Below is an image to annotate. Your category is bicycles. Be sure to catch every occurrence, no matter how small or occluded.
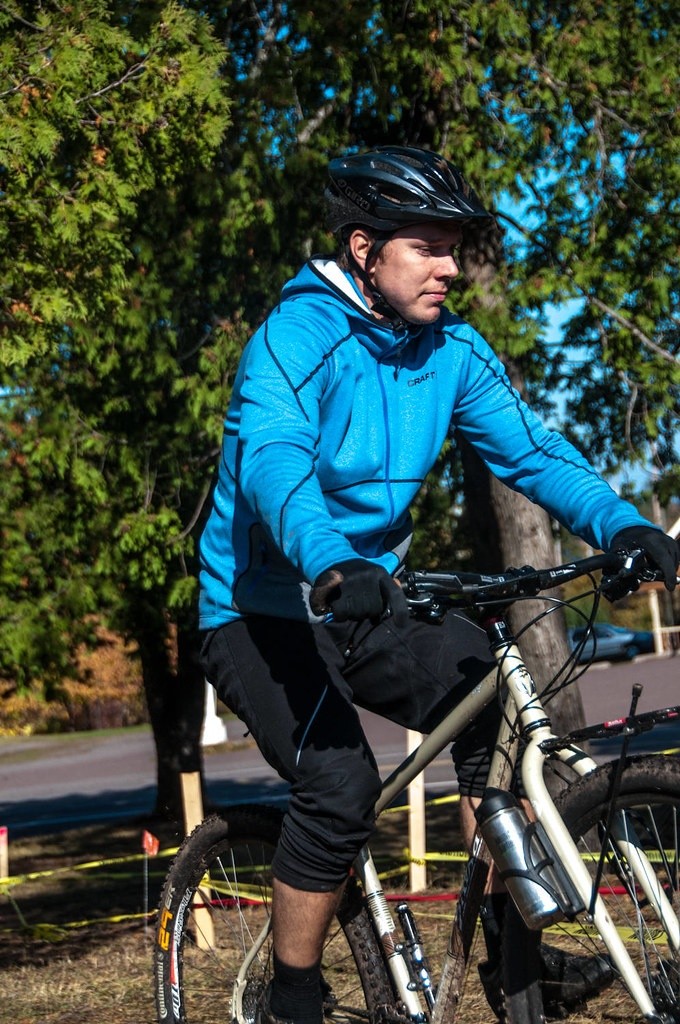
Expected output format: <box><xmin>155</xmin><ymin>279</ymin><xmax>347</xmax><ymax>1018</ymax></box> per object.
<box><xmin>154</xmin><ymin>540</ymin><xmax>680</xmax><ymax>1023</ymax></box>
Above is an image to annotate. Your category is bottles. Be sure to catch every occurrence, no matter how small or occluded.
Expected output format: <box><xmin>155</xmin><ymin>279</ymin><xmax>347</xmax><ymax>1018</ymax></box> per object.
<box><xmin>474</xmin><ymin>787</ymin><xmax>565</xmax><ymax>930</ymax></box>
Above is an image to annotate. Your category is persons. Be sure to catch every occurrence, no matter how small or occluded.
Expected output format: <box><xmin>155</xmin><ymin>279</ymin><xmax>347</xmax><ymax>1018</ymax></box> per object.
<box><xmin>197</xmin><ymin>147</ymin><xmax>680</xmax><ymax>1024</ymax></box>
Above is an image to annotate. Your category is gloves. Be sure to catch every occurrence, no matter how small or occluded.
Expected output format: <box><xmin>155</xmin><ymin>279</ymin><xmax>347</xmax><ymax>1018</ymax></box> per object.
<box><xmin>325</xmin><ymin>558</ymin><xmax>408</xmax><ymax>629</ymax></box>
<box><xmin>603</xmin><ymin>527</ymin><xmax>680</xmax><ymax>593</ymax></box>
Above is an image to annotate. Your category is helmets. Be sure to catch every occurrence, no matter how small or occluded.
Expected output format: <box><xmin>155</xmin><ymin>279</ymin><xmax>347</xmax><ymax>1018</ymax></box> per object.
<box><xmin>326</xmin><ymin>146</ymin><xmax>494</xmax><ymax>238</ymax></box>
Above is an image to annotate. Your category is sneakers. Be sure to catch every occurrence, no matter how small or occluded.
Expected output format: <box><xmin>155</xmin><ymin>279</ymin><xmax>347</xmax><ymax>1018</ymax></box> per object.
<box><xmin>478</xmin><ymin>943</ymin><xmax>615</xmax><ymax>1024</ymax></box>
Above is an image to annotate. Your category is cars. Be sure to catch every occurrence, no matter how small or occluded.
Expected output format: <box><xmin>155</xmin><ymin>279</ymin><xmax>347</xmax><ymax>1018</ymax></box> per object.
<box><xmin>567</xmin><ymin>622</ymin><xmax>654</xmax><ymax>665</ymax></box>
